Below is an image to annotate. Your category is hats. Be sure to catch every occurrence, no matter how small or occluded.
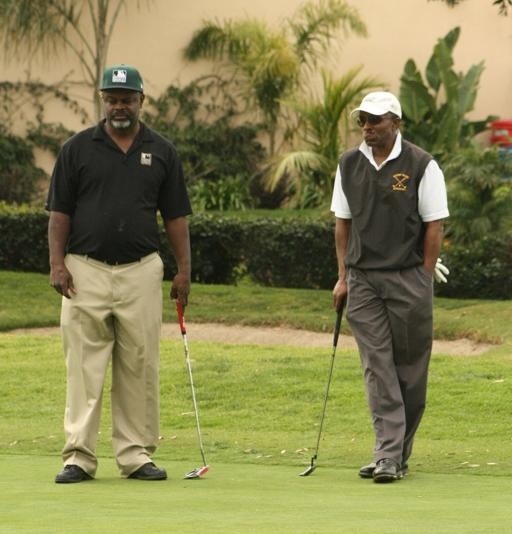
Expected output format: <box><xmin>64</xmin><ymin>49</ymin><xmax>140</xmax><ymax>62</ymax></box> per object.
<box><xmin>100</xmin><ymin>64</ymin><xmax>143</xmax><ymax>94</ymax></box>
<box><xmin>350</xmin><ymin>90</ymin><xmax>402</xmax><ymax>120</ymax></box>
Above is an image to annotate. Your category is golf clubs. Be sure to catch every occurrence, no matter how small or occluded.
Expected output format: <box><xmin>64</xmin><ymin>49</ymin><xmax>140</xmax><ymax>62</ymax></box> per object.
<box><xmin>176</xmin><ymin>297</ymin><xmax>209</xmax><ymax>479</ymax></box>
<box><xmin>300</xmin><ymin>294</ymin><xmax>345</xmax><ymax>476</ymax></box>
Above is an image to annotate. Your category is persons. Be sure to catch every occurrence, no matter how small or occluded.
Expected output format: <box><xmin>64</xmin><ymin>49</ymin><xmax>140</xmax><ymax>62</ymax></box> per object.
<box><xmin>44</xmin><ymin>63</ymin><xmax>193</xmax><ymax>484</ymax></box>
<box><xmin>329</xmin><ymin>91</ymin><xmax>450</xmax><ymax>484</ymax></box>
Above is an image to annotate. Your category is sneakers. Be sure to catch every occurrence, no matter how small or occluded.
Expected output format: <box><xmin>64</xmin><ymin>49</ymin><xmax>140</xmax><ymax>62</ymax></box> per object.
<box><xmin>359</xmin><ymin>463</ymin><xmax>408</xmax><ymax>478</ymax></box>
<box><xmin>373</xmin><ymin>459</ymin><xmax>405</xmax><ymax>482</ymax></box>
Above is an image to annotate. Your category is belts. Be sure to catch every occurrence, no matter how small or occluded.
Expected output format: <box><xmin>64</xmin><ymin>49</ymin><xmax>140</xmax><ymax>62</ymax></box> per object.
<box><xmin>84</xmin><ymin>249</ymin><xmax>161</xmax><ymax>267</ymax></box>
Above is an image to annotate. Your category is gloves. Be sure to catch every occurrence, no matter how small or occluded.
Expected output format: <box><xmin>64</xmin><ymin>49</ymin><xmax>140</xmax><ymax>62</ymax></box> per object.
<box><xmin>433</xmin><ymin>257</ymin><xmax>449</xmax><ymax>285</ymax></box>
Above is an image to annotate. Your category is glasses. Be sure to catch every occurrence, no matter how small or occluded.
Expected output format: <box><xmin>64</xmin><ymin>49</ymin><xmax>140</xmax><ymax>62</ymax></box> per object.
<box><xmin>356</xmin><ymin>113</ymin><xmax>394</xmax><ymax>126</ymax></box>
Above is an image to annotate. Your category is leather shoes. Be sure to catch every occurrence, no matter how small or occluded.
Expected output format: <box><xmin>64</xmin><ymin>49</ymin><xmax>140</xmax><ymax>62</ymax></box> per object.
<box><xmin>54</xmin><ymin>465</ymin><xmax>94</xmax><ymax>484</ymax></box>
<box><xmin>127</xmin><ymin>462</ymin><xmax>167</xmax><ymax>480</ymax></box>
